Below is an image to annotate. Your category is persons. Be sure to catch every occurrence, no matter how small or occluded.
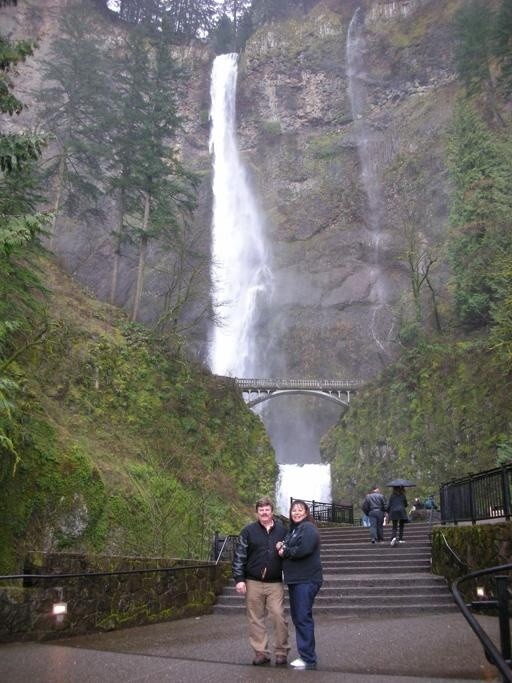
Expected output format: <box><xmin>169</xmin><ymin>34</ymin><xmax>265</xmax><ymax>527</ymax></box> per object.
<box><xmin>274</xmin><ymin>498</ymin><xmax>324</xmax><ymax>667</ymax></box>
<box><xmin>360</xmin><ymin>485</ymin><xmax>387</xmax><ymax>544</ymax></box>
<box><xmin>231</xmin><ymin>495</ymin><xmax>290</xmax><ymax>666</ymax></box>
<box><xmin>384</xmin><ymin>485</ymin><xmax>410</xmax><ymax>547</ymax></box>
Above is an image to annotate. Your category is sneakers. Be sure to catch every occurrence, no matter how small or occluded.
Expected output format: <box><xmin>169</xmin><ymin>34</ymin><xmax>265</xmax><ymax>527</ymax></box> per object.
<box><xmin>252</xmin><ymin>652</ymin><xmax>271</xmax><ymax>666</ymax></box>
<box><xmin>372</xmin><ymin>537</ymin><xmax>407</xmax><ymax>546</ymax></box>
<box><xmin>275</xmin><ymin>652</ymin><xmax>287</xmax><ymax>666</ymax></box>
<box><xmin>289</xmin><ymin>655</ymin><xmax>317</xmax><ymax>667</ymax></box>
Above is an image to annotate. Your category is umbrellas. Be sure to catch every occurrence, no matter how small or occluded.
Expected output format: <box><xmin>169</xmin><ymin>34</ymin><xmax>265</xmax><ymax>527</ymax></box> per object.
<box><xmin>384</xmin><ymin>478</ymin><xmax>416</xmax><ymax>489</ymax></box>
